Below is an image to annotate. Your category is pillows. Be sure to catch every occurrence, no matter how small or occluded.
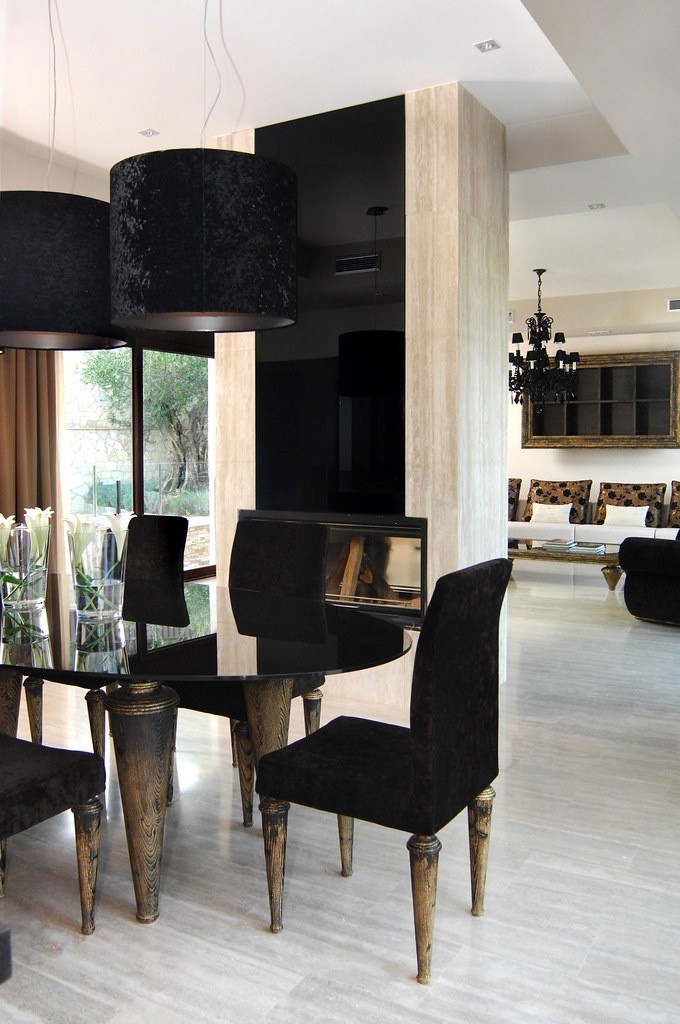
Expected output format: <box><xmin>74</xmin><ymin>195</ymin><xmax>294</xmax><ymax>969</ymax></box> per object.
<box><xmin>602</xmin><ymin>504</ymin><xmax>649</xmax><ymax>527</ymax></box>
<box><xmin>529</xmin><ymin>503</ymin><xmax>572</xmax><ymax>524</ymax></box>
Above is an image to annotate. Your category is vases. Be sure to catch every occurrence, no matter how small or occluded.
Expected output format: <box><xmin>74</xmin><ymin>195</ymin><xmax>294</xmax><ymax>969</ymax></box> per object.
<box><xmin>0</xmin><ymin>523</ymin><xmax>51</xmax><ymax>605</ymax></box>
<box><xmin>66</xmin><ymin>528</ymin><xmax>128</xmax><ymax>617</ymax></box>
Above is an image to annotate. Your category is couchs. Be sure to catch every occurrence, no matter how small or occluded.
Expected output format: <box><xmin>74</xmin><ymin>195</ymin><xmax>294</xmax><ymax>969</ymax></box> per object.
<box><xmin>619</xmin><ymin>529</ymin><xmax>680</xmax><ymax>628</ymax></box>
<box><xmin>508</xmin><ymin>478</ymin><xmax>680</xmax><ymax>544</ymax></box>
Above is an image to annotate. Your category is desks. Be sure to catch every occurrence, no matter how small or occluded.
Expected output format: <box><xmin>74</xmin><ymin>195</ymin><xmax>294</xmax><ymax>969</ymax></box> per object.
<box><xmin>0</xmin><ymin>579</ymin><xmax>411</xmax><ymax>933</ymax></box>
<box><xmin>508</xmin><ymin>538</ymin><xmax>624</xmax><ymax>590</ymax></box>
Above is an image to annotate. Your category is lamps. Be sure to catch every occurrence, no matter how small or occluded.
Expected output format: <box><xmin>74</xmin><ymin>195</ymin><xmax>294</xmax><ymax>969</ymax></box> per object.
<box><xmin>110</xmin><ymin>0</ymin><xmax>295</xmax><ymax>331</ymax></box>
<box><xmin>0</xmin><ymin>0</ymin><xmax>130</xmax><ymax>350</ymax></box>
<box><xmin>337</xmin><ymin>206</ymin><xmax>406</xmax><ymax>397</ymax></box>
<box><xmin>509</xmin><ymin>269</ymin><xmax>580</xmax><ymax>412</ymax></box>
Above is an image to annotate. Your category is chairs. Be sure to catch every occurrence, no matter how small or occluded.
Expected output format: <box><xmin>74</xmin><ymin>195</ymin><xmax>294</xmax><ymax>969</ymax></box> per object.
<box><xmin>0</xmin><ymin>515</ymin><xmax>511</xmax><ymax>984</ymax></box>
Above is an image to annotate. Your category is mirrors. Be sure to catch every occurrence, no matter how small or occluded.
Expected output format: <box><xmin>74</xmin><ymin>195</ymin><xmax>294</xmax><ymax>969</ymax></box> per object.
<box><xmin>254</xmin><ymin>93</ymin><xmax>405</xmax><ymax>515</ymax></box>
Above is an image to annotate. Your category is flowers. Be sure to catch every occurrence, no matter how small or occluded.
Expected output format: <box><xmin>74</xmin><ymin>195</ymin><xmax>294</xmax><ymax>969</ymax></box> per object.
<box><xmin>0</xmin><ymin>506</ymin><xmax>55</xmax><ymax>604</ymax></box>
<box><xmin>62</xmin><ymin>509</ymin><xmax>137</xmax><ymax>616</ymax></box>
